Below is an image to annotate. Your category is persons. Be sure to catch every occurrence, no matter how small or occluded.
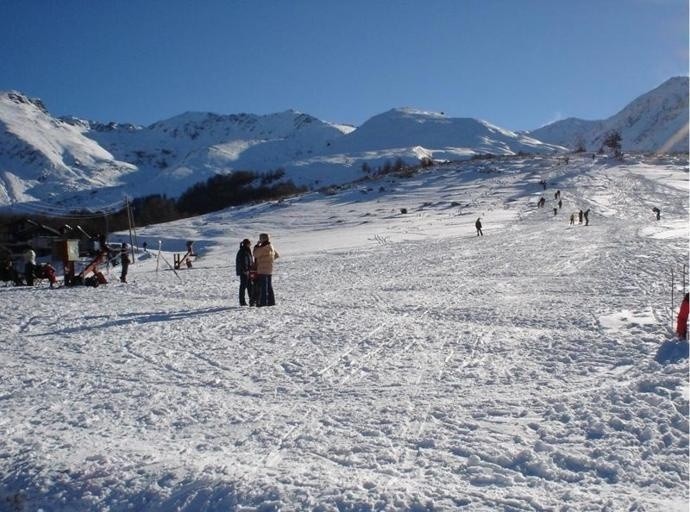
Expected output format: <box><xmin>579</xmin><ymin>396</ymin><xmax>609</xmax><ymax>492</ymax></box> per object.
<box><xmin>120</xmin><ymin>242</ymin><xmax>131</xmax><ymax>283</ymax></box>
<box><xmin>652</xmin><ymin>207</ymin><xmax>660</xmax><ymax>222</ymax></box>
<box><xmin>475</xmin><ymin>217</ymin><xmax>483</xmax><ymax>237</ymax></box>
<box><xmin>535</xmin><ymin>176</ymin><xmax>591</xmax><ymax>227</ymax></box>
<box><xmin>252</xmin><ymin>232</ymin><xmax>280</xmax><ymax>307</ymax></box>
<box><xmin>674</xmin><ymin>290</ymin><xmax>689</xmax><ymax>343</ymax></box>
<box><xmin>235</xmin><ymin>238</ymin><xmax>256</xmax><ymax>307</ymax></box>
<box><xmin>43</xmin><ymin>262</ymin><xmax>62</xmax><ymax>289</ymax></box>
